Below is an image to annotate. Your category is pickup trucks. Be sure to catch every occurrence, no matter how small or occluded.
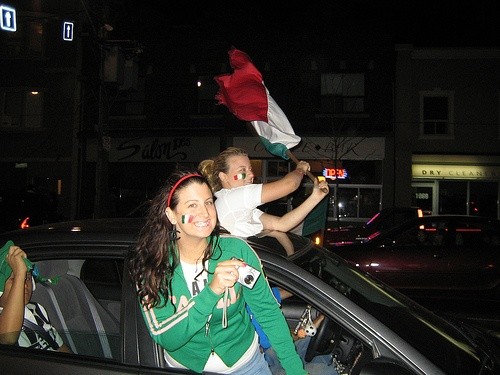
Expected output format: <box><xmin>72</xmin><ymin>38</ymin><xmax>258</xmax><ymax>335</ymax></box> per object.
<box><xmin>314</xmin><ymin>207</ymin><xmax>486</xmax><ymax>248</ymax></box>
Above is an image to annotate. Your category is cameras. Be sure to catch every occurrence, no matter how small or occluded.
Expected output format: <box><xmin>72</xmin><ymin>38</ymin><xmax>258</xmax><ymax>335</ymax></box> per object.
<box><xmin>228</xmin><ymin>256</ymin><xmax>261</xmax><ymax>290</ymax></box>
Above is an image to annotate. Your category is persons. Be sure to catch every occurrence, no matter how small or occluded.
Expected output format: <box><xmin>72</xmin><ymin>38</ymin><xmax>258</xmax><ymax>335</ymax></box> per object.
<box><xmin>0</xmin><ymin>240</ymin><xmax>73</xmax><ymax>353</ymax></box>
<box><xmin>245</xmin><ymin>287</ymin><xmax>338</xmax><ymax>375</ymax></box>
<box><xmin>128</xmin><ymin>169</ymin><xmax>307</xmax><ymax>375</ymax></box>
<box><xmin>221</xmin><ymin>225</ymin><xmax>295</xmax><ymax>257</ymax></box>
<box><xmin>198</xmin><ymin>147</ymin><xmax>329</xmax><ymax>232</ymax></box>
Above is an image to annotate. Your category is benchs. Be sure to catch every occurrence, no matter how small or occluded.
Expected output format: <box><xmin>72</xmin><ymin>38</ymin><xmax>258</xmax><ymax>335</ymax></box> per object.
<box><xmin>31</xmin><ymin>275</ymin><xmax>121</xmax><ymax>359</ymax></box>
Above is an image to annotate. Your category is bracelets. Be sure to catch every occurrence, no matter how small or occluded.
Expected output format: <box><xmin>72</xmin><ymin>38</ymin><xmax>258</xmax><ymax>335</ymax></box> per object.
<box><xmin>295</xmin><ymin>169</ymin><xmax>305</xmax><ymax>175</ymax></box>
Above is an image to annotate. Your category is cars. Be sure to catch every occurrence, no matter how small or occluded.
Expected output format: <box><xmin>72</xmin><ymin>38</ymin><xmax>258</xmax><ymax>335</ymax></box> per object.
<box><xmin>0</xmin><ymin>217</ymin><xmax>500</xmax><ymax>375</ymax></box>
<box><xmin>323</xmin><ymin>214</ymin><xmax>500</xmax><ymax>308</ymax></box>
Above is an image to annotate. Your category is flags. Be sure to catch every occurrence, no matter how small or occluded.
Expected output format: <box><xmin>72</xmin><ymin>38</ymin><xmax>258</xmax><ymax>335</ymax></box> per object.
<box><xmin>287</xmin><ymin>192</ymin><xmax>329</xmax><ymax>236</ymax></box>
<box><xmin>214</xmin><ymin>51</ymin><xmax>302</xmax><ymax>159</ymax></box>
